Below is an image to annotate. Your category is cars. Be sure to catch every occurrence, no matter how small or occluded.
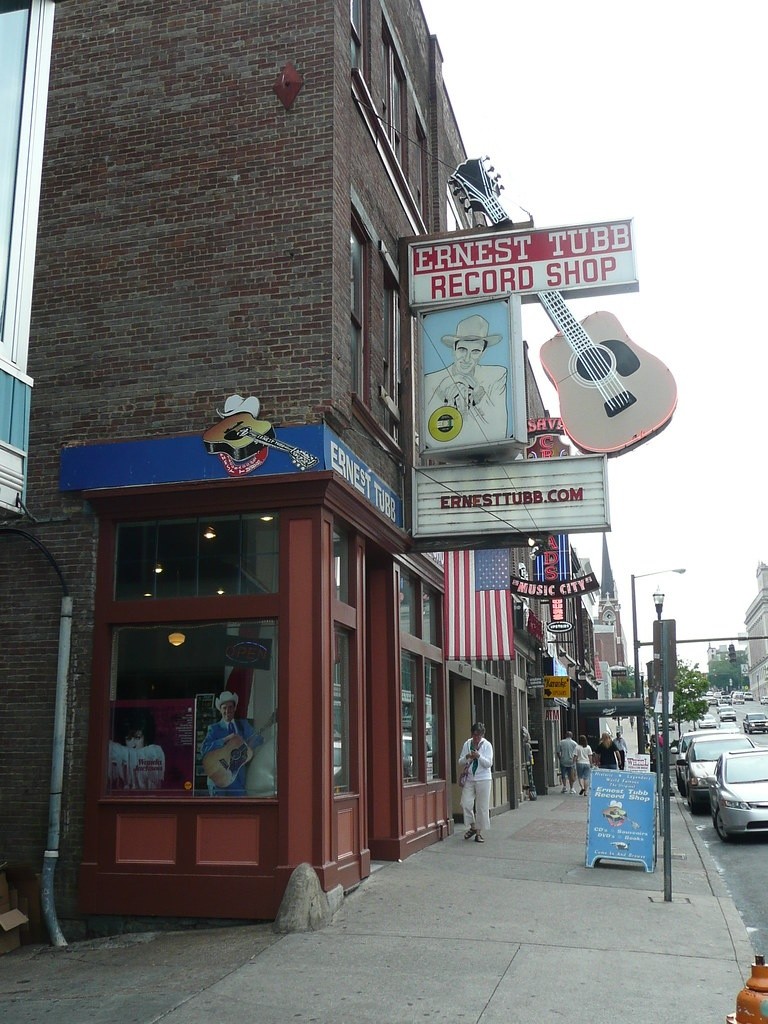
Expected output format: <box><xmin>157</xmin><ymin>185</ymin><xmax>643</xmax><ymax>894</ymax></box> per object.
<box><xmin>717</xmin><ymin>722</ymin><xmax>742</xmax><ymax>734</ymax></box>
<box><xmin>705</xmin><ymin>747</ymin><xmax>768</xmax><ymax>845</ymax></box>
<box><xmin>698</xmin><ymin>714</ymin><xmax>717</xmax><ymax>729</ymax></box>
<box><xmin>671</xmin><ymin>729</ymin><xmax>736</xmax><ymax>798</ymax></box>
<box><xmin>676</xmin><ymin>733</ymin><xmax>762</xmax><ymax>816</ymax></box>
<box><xmin>332</xmin><ymin>742</ymin><xmax>346</xmax><ymax>794</ymax></box>
<box><xmin>706</xmin><ymin>691</ymin><xmax>714</xmax><ymax>697</ymax></box>
<box><xmin>707</xmin><ymin>698</ymin><xmax>718</xmax><ymax>707</ymax></box>
<box><xmin>401</xmin><ymin>735</ymin><xmax>436</xmax><ymax>786</ymax></box>
<box><xmin>719</xmin><ymin>706</ymin><xmax>736</xmax><ymax>721</ymax></box>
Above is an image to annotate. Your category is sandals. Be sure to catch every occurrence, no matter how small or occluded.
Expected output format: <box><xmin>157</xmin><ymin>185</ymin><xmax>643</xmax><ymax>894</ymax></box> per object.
<box><xmin>475</xmin><ymin>834</ymin><xmax>485</xmax><ymax>843</ymax></box>
<box><xmin>463</xmin><ymin>827</ymin><xmax>477</xmax><ymax>840</ymax></box>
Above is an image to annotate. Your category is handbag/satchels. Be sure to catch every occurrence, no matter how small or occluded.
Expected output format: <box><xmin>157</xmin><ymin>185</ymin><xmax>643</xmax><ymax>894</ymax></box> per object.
<box><xmin>457</xmin><ymin>765</ymin><xmax>469</xmax><ymax>788</ymax></box>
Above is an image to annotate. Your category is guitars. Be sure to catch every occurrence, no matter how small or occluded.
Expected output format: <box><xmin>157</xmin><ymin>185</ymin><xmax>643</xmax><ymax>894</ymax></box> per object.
<box><xmin>448</xmin><ymin>156</ymin><xmax>676</xmax><ymax>459</ymax></box>
<box><xmin>203</xmin><ymin>411</ymin><xmax>318</xmax><ymax>470</ymax></box>
<box><xmin>202</xmin><ymin>704</ymin><xmax>279</xmax><ymax>788</ymax></box>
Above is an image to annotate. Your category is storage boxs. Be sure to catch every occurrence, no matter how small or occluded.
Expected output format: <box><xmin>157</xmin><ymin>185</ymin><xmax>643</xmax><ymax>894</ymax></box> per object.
<box><xmin>0</xmin><ymin>863</ymin><xmax>43</xmax><ymax>952</ymax></box>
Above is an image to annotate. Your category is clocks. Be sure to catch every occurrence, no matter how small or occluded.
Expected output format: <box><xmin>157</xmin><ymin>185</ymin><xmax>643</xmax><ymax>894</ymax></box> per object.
<box><xmin>603</xmin><ymin>609</ymin><xmax>616</xmax><ymax>622</ymax></box>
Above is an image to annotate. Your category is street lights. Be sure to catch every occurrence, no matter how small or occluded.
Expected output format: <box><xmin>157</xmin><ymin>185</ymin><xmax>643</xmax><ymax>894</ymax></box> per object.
<box><xmin>628</xmin><ymin>567</ymin><xmax>688</xmax><ymax>755</ymax></box>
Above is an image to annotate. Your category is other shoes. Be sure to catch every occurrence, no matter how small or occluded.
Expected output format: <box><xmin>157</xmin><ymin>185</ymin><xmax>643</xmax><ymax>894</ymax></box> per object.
<box><xmin>578</xmin><ymin>789</ymin><xmax>587</xmax><ymax>796</ymax></box>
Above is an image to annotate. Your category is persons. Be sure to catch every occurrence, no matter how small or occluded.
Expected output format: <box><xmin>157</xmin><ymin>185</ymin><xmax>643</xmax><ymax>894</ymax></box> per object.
<box><xmin>556</xmin><ymin>730</ymin><xmax>577</xmax><ymax>794</ymax></box>
<box><xmin>612</xmin><ymin>731</ymin><xmax>628</xmax><ymax>770</ymax></box>
<box><xmin>572</xmin><ymin>735</ymin><xmax>594</xmax><ymax>796</ymax></box>
<box><xmin>630</xmin><ymin>715</ymin><xmax>636</xmax><ymax>731</ymax></box>
<box><xmin>644</xmin><ymin>731</ymin><xmax>664</xmax><ymax>755</ymax></box>
<box><xmin>120</xmin><ymin>709</ymin><xmax>173</xmax><ymax>790</ymax></box>
<box><xmin>201</xmin><ymin>692</ymin><xmax>264</xmax><ymax>795</ymax></box>
<box><xmin>422</xmin><ymin>314</ymin><xmax>507</xmax><ymax>443</ymax></box>
<box><xmin>458</xmin><ymin>722</ymin><xmax>494</xmax><ymax>844</ymax></box>
<box><xmin>595</xmin><ymin>731</ymin><xmax>622</xmax><ymax>770</ymax></box>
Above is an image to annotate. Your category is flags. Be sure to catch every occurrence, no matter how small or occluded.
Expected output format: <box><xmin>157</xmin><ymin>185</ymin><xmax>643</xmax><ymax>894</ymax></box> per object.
<box><xmin>654</xmin><ymin>691</ymin><xmax>675</xmax><ymax>715</ymax></box>
<box><xmin>443</xmin><ymin>548</ymin><xmax>515</xmax><ymax>662</ymax></box>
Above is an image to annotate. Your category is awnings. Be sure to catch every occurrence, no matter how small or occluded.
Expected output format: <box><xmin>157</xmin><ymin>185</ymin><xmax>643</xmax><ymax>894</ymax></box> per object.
<box><xmin>578</xmin><ymin>697</ymin><xmax>644</xmax><ymax>718</ymax></box>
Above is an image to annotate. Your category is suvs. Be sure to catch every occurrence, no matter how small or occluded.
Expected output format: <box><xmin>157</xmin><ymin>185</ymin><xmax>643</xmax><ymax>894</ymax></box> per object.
<box><xmin>714</xmin><ymin>692</ymin><xmax>722</xmax><ymax>698</ymax></box>
<box><xmin>743</xmin><ymin>713</ymin><xmax>768</xmax><ymax>734</ymax></box>
<box><xmin>760</xmin><ymin>696</ymin><xmax>768</xmax><ymax>705</ymax></box>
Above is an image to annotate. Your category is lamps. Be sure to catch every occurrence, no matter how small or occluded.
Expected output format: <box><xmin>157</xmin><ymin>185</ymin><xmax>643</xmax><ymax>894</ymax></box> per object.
<box><xmin>203</xmin><ymin>526</ymin><xmax>217</xmax><ymax>539</ymax></box>
<box><xmin>155</xmin><ymin>564</ymin><xmax>162</xmax><ymax>572</ymax></box>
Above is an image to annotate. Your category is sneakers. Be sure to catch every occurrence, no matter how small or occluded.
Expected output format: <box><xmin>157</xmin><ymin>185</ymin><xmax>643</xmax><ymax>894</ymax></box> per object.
<box><xmin>561</xmin><ymin>788</ymin><xmax>568</xmax><ymax>793</ymax></box>
<box><xmin>570</xmin><ymin>789</ymin><xmax>577</xmax><ymax>794</ymax></box>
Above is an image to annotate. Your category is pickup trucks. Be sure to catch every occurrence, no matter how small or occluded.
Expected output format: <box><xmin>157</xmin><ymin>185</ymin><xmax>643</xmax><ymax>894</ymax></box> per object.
<box><xmin>721</xmin><ymin>695</ymin><xmax>732</xmax><ymax>705</ymax></box>
<box><xmin>743</xmin><ymin>692</ymin><xmax>754</xmax><ymax>701</ymax></box>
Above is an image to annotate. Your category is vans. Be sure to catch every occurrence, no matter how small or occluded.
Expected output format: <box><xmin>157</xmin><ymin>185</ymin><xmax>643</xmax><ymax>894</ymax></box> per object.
<box><xmin>732</xmin><ymin>692</ymin><xmax>745</xmax><ymax>705</ymax></box>
